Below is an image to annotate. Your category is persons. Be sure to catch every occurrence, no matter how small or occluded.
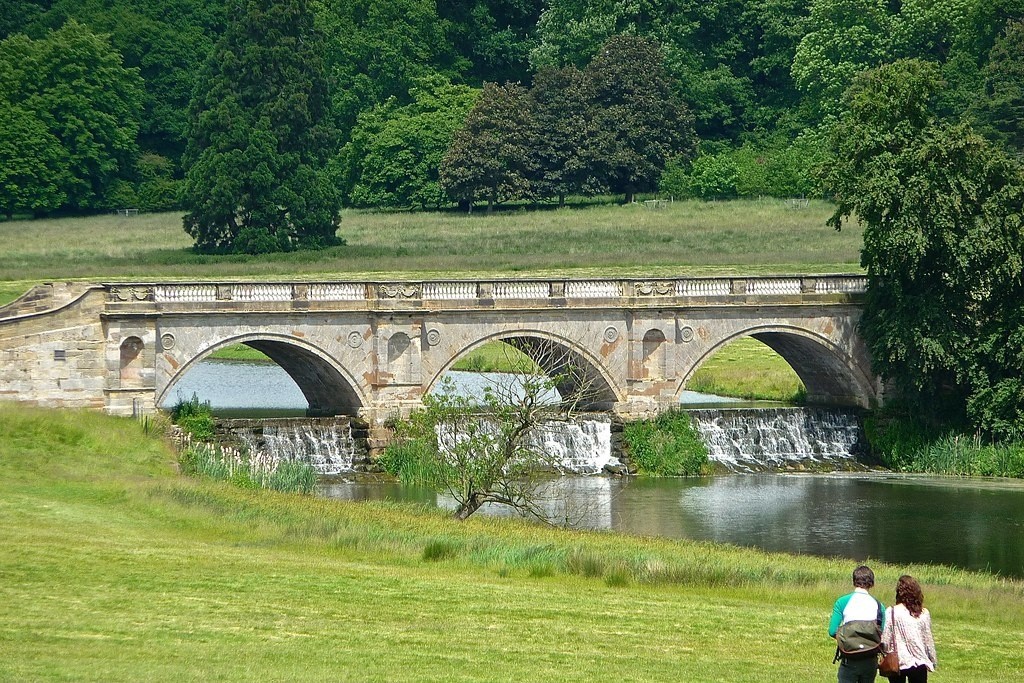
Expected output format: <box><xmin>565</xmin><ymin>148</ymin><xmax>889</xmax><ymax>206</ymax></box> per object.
<box><xmin>828</xmin><ymin>565</ymin><xmax>886</xmax><ymax>683</ymax></box>
<box><xmin>880</xmin><ymin>574</ymin><xmax>938</xmax><ymax>683</ymax></box>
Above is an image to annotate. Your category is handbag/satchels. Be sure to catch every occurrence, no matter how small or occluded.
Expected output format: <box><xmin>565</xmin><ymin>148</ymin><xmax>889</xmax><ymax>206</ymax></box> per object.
<box><xmin>878</xmin><ymin>651</ymin><xmax>899</xmax><ymax>678</ymax></box>
<box><xmin>833</xmin><ymin>620</ymin><xmax>882</xmax><ymax>664</ymax></box>
